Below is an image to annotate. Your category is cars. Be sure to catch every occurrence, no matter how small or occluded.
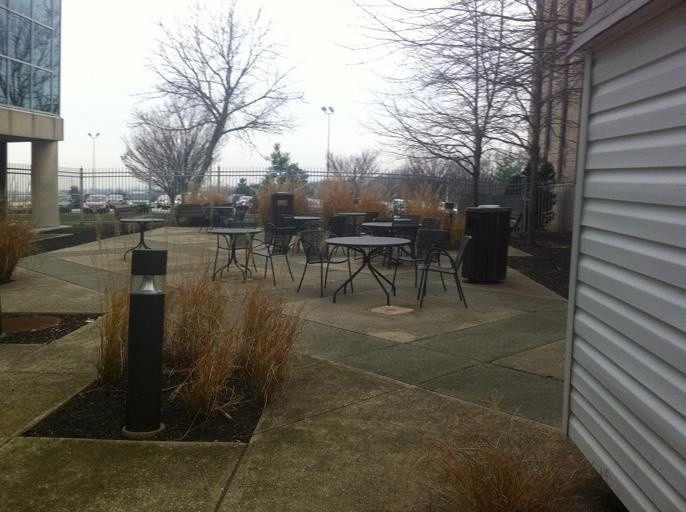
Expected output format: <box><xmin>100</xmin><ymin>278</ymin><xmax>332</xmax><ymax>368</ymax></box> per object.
<box><xmin>9</xmin><ymin>194</ymin><xmax>33</xmax><ymax>213</ymax></box>
<box><xmin>58</xmin><ymin>192</ymin><xmax>256</xmax><ymax>213</ymax></box>
<box><xmin>305</xmin><ymin>198</ymin><xmax>458</xmax><ymax>213</ymax></box>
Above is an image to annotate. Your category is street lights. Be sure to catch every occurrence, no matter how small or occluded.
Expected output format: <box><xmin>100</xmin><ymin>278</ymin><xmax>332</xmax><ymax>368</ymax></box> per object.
<box><xmin>321</xmin><ymin>105</ymin><xmax>335</xmax><ymax>182</ymax></box>
<box><xmin>87</xmin><ymin>132</ymin><xmax>100</xmax><ymax>193</ymax></box>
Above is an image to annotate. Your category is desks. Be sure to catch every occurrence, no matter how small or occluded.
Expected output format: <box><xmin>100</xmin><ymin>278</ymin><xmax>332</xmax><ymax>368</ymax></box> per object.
<box><xmin>119</xmin><ymin>218</ymin><xmax>165</xmax><ymax>260</ymax></box>
<box><xmin>206</xmin><ymin>228</ymin><xmax>265</xmax><ymax>285</ymax></box>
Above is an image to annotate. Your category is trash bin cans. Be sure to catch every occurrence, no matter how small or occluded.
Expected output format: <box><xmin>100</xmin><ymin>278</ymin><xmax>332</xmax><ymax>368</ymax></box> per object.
<box><xmin>271</xmin><ymin>191</ymin><xmax>295</xmax><ymax>226</ymax></box>
<box><xmin>461</xmin><ymin>204</ymin><xmax>512</xmax><ymax>283</ymax></box>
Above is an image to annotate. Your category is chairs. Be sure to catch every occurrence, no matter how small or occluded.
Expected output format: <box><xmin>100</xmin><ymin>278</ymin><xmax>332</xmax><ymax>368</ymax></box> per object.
<box><xmin>213</xmin><ymin>205</ymin><xmax>474</xmax><ymax>310</ymax></box>
